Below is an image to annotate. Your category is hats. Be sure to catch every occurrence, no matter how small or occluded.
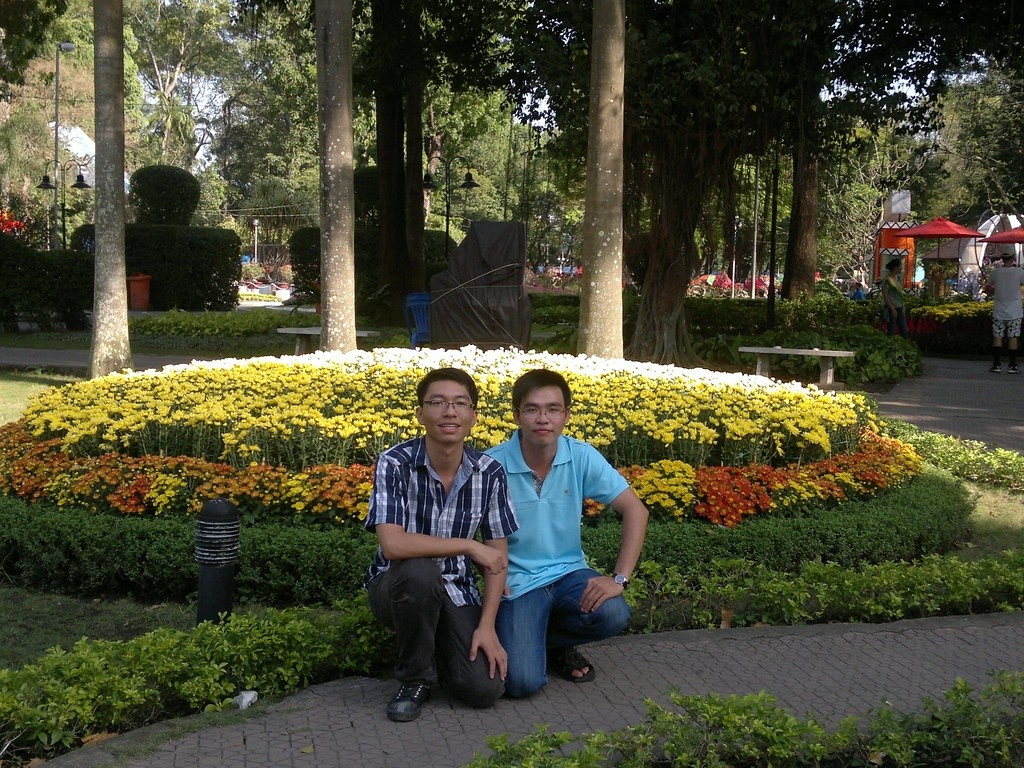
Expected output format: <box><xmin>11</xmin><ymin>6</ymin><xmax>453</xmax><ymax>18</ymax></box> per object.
<box><xmin>1001</xmin><ymin>244</ymin><xmax>1015</xmax><ymax>256</ymax></box>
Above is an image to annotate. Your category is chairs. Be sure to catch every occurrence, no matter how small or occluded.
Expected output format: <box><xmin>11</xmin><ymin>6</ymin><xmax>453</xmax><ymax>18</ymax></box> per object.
<box><xmin>404</xmin><ymin>293</ymin><xmax>431</xmax><ymax>349</ymax></box>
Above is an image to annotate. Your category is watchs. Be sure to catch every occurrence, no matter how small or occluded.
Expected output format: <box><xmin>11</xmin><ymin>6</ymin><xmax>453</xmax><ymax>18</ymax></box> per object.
<box><xmin>612</xmin><ymin>573</ymin><xmax>627</xmax><ymax>590</ymax></box>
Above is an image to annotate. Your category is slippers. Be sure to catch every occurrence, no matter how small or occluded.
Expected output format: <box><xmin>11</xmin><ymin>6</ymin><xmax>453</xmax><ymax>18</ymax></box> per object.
<box><xmin>547</xmin><ymin>647</ymin><xmax>595</xmax><ymax>683</ymax></box>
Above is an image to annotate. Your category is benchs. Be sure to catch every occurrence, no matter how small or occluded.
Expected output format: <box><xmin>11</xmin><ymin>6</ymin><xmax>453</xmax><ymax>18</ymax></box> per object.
<box><xmin>739</xmin><ymin>345</ymin><xmax>856</xmax><ymax>390</ymax></box>
<box><xmin>276</xmin><ymin>327</ymin><xmax>380</xmax><ymax>355</ymax></box>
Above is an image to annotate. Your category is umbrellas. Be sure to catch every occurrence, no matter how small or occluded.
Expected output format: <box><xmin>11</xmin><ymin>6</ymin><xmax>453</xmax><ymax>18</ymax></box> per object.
<box><xmin>978</xmin><ymin>226</ymin><xmax>1024</xmax><ymax>244</ymax></box>
<box><xmin>895</xmin><ymin>217</ymin><xmax>986</xmax><ymax>263</ymax></box>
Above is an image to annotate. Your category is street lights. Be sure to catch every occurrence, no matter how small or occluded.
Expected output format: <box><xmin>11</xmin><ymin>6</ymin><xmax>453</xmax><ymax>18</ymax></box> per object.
<box><xmin>53</xmin><ymin>43</ymin><xmax>75</xmax><ymax>252</ymax></box>
<box><xmin>422</xmin><ymin>155</ymin><xmax>481</xmax><ymax>260</ymax></box>
<box><xmin>36</xmin><ymin>160</ymin><xmax>93</xmax><ymax>252</ymax></box>
<box><xmin>732</xmin><ymin>214</ymin><xmax>739</xmax><ymax>299</ymax></box>
<box><xmin>253</xmin><ymin>219</ymin><xmax>259</xmax><ymax>262</ymax></box>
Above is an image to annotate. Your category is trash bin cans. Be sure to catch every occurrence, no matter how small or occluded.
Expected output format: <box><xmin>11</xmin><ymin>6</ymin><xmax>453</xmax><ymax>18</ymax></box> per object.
<box><xmin>127</xmin><ymin>272</ymin><xmax>152</xmax><ymax>311</ymax></box>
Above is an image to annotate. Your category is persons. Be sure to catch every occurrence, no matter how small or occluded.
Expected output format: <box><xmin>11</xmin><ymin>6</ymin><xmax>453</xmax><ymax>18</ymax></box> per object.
<box><xmin>883</xmin><ymin>258</ymin><xmax>907</xmax><ymax>340</ymax></box>
<box><xmin>985</xmin><ymin>246</ymin><xmax>1024</xmax><ymax>374</ymax></box>
<box><xmin>852</xmin><ymin>283</ymin><xmax>863</xmax><ymax>300</ymax></box>
<box><xmin>481</xmin><ymin>370</ymin><xmax>650</xmax><ymax>697</ymax></box>
<box><xmin>363</xmin><ymin>369</ymin><xmax>521</xmax><ymax>720</ymax></box>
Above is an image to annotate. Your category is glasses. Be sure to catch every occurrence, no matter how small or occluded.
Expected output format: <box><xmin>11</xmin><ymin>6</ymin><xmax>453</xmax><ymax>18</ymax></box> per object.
<box><xmin>515</xmin><ymin>408</ymin><xmax>568</xmax><ymax>418</ymax></box>
<box><xmin>423</xmin><ymin>400</ymin><xmax>474</xmax><ymax>413</ymax></box>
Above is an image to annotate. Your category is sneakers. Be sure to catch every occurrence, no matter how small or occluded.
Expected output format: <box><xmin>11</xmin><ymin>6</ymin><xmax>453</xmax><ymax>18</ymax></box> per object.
<box><xmin>387</xmin><ymin>684</ymin><xmax>431</xmax><ymax>721</ymax></box>
<box><xmin>1007</xmin><ymin>364</ymin><xmax>1020</xmax><ymax>373</ymax></box>
<box><xmin>990</xmin><ymin>366</ymin><xmax>1000</xmax><ymax>372</ymax></box>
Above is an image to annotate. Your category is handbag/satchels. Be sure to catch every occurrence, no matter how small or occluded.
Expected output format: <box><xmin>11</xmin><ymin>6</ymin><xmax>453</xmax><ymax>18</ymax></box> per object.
<box><xmin>879</xmin><ymin>306</ymin><xmax>891</xmax><ymax>322</ymax></box>
